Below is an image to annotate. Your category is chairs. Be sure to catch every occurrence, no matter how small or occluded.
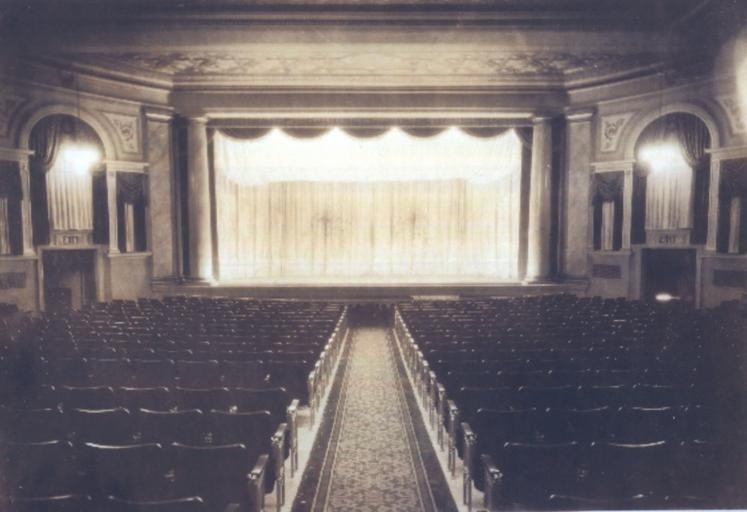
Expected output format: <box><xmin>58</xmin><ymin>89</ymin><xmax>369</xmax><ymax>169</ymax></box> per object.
<box><xmin>1</xmin><ymin>294</ymin><xmax>348</xmax><ymax>512</ymax></box>
<box><xmin>394</xmin><ymin>292</ymin><xmax>747</xmax><ymax>511</ymax></box>
